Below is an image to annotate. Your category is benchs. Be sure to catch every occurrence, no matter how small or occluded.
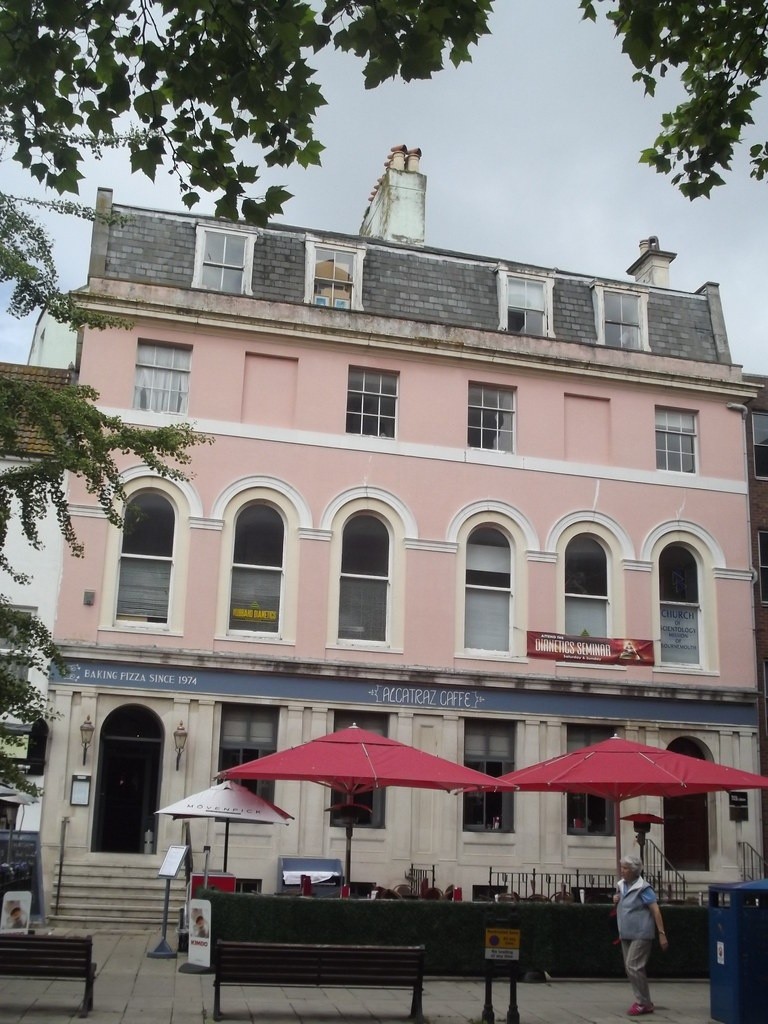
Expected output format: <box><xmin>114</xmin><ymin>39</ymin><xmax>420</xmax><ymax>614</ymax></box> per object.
<box><xmin>0</xmin><ymin>931</ymin><xmax>97</xmax><ymax>1019</ymax></box>
<box><xmin>211</xmin><ymin>938</ymin><xmax>425</xmax><ymax>1024</ymax></box>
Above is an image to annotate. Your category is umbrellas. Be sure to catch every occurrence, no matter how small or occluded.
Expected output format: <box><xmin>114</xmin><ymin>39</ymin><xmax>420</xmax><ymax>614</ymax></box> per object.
<box><xmin>453</xmin><ymin>733</ymin><xmax>768</xmax><ymax>896</ymax></box>
<box><xmin>153</xmin><ymin>780</ymin><xmax>295</xmax><ymax>873</ymax></box>
<box><xmin>211</xmin><ymin>722</ymin><xmax>520</xmax><ymax>887</ymax></box>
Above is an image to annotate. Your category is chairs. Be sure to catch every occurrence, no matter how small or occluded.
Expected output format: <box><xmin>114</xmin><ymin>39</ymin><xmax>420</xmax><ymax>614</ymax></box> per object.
<box><xmin>376</xmin><ymin>883</ymin><xmax>606</xmax><ymax>904</ymax></box>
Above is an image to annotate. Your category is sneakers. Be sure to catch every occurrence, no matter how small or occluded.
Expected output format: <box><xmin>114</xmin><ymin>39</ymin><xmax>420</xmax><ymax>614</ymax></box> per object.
<box><xmin>627</xmin><ymin>1001</ymin><xmax>654</xmax><ymax>1015</ymax></box>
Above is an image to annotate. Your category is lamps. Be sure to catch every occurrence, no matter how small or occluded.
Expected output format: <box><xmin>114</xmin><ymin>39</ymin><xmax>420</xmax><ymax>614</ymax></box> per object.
<box><xmin>80</xmin><ymin>714</ymin><xmax>95</xmax><ymax>767</ymax></box>
<box><xmin>172</xmin><ymin>720</ymin><xmax>188</xmax><ymax>771</ymax></box>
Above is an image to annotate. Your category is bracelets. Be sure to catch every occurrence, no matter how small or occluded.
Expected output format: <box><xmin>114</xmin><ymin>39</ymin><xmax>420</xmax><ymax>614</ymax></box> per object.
<box><xmin>659</xmin><ymin>931</ymin><xmax>665</xmax><ymax>935</ymax></box>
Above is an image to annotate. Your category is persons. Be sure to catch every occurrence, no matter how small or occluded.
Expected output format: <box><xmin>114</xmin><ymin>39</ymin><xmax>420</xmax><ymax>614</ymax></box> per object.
<box><xmin>613</xmin><ymin>856</ymin><xmax>668</xmax><ymax>1015</ymax></box>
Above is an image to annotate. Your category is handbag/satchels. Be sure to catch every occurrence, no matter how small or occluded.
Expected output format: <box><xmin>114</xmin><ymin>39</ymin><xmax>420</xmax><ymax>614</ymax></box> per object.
<box><xmin>608</xmin><ymin>902</ymin><xmax>620</xmax><ymax>944</ymax></box>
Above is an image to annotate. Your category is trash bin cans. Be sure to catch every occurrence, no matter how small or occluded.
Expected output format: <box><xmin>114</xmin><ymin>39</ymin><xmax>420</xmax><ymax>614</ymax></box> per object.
<box><xmin>708</xmin><ymin>879</ymin><xmax>768</xmax><ymax>1024</ymax></box>
